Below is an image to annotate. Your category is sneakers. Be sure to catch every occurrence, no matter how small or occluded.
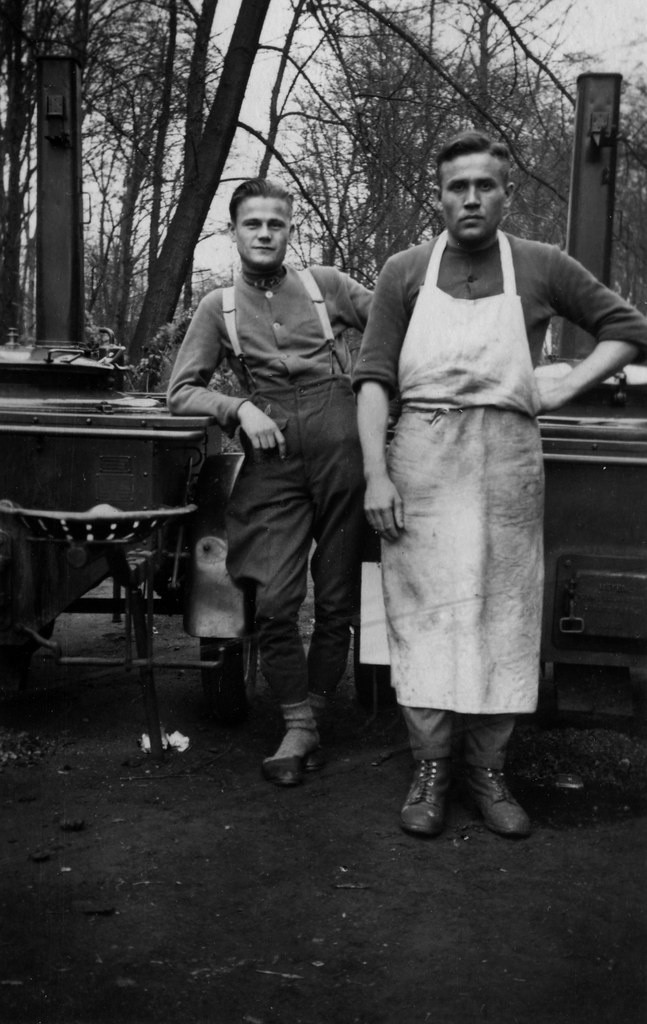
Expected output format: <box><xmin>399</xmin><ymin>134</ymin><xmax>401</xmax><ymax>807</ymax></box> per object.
<box><xmin>462</xmin><ymin>760</ymin><xmax>530</xmax><ymax>837</ymax></box>
<box><xmin>401</xmin><ymin>758</ymin><xmax>451</xmax><ymax>836</ymax></box>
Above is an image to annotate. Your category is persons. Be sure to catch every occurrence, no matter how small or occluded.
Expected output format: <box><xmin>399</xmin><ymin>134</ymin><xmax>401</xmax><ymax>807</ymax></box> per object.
<box><xmin>166</xmin><ymin>178</ymin><xmax>374</xmax><ymax>784</ymax></box>
<box><xmin>356</xmin><ymin>131</ymin><xmax>647</xmax><ymax>835</ymax></box>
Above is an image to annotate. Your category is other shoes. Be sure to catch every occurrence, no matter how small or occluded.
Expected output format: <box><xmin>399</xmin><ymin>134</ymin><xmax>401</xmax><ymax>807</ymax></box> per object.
<box><xmin>273</xmin><ymin>767</ymin><xmax>300</xmax><ymax>786</ymax></box>
<box><xmin>301</xmin><ymin>715</ymin><xmax>335</xmax><ymax>774</ymax></box>
